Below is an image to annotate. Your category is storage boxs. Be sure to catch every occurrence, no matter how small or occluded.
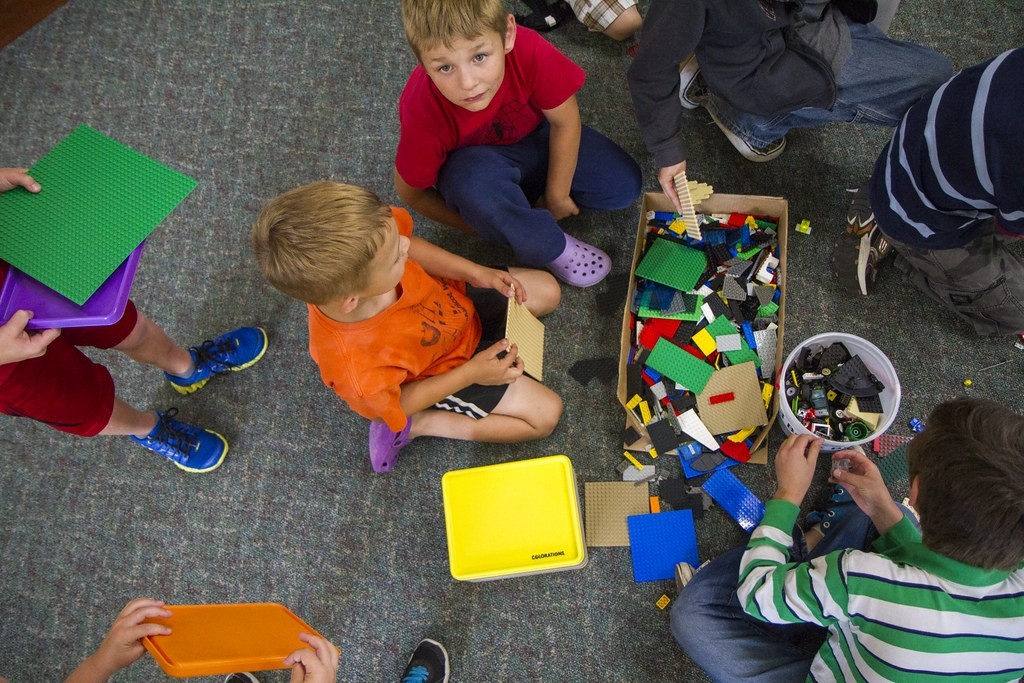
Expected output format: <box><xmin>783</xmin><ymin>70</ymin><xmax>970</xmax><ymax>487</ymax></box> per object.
<box><xmin>615</xmin><ymin>192</ymin><xmax>788</xmax><ymax>466</ymax></box>
<box><xmin>440</xmin><ymin>453</ymin><xmax>587</xmax><ymax>583</ymax></box>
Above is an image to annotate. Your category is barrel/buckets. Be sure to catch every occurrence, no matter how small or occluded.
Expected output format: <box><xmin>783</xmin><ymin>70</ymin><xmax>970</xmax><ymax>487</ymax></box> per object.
<box><xmin>776</xmin><ymin>330</ymin><xmax>902</xmax><ymax>454</ymax></box>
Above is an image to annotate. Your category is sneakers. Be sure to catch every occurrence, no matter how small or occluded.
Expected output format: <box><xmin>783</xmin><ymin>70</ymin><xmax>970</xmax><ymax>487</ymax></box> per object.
<box><xmin>831</xmin><ymin>182</ymin><xmax>892</xmax><ymax>297</ymax></box>
<box><xmin>805</xmin><ymin>445</ymin><xmax>867</xmax><ymax>536</ymax></box>
<box><xmin>129</xmin><ymin>407</ymin><xmax>228</xmax><ymax>473</ymax></box>
<box><xmin>164</xmin><ymin>326</ymin><xmax>268</xmax><ymax>395</ymax></box>
<box><xmin>224</xmin><ymin>672</ymin><xmax>260</xmax><ymax>683</ymax></box>
<box><xmin>678</xmin><ymin>51</ymin><xmax>786</xmax><ymax>162</ymax></box>
<box><xmin>398</xmin><ymin>638</ymin><xmax>450</xmax><ymax>683</ymax></box>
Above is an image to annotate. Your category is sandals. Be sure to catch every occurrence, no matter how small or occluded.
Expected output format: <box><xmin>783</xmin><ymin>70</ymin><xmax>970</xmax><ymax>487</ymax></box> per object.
<box><xmin>544</xmin><ymin>232</ymin><xmax>612</xmax><ymax>288</ymax></box>
<box><xmin>369</xmin><ymin>412</ymin><xmax>413</xmax><ymax>473</ymax></box>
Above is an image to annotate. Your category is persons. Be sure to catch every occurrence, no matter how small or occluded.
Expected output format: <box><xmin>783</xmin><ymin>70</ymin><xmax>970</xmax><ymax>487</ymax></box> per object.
<box><xmin>394</xmin><ymin>0</ymin><xmax>642</xmax><ymax>287</ymax></box>
<box><xmin>563</xmin><ymin>0</ymin><xmax>952</xmax><ymax>216</ymax></box>
<box><xmin>847</xmin><ymin>46</ymin><xmax>1024</xmax><ymax>337</ymax></box>
<box><xmin>0</xmin><ymin>168</ymin><xmax>269</xmax><ymax>473</ymax></box>
<box><xmin>670</xmin><ymin>398</ymin><xmax>1024</xmax><ymax>683</ymax></box>
<box><xmin>250</xmin><ymin>183</ymin><xmax>560</xmax><ymax>473</ymax></box>
<box><xmin>64</xmin><ymin>597</ymin><xmax>450</xmax><ymax>683</ymax></box>
